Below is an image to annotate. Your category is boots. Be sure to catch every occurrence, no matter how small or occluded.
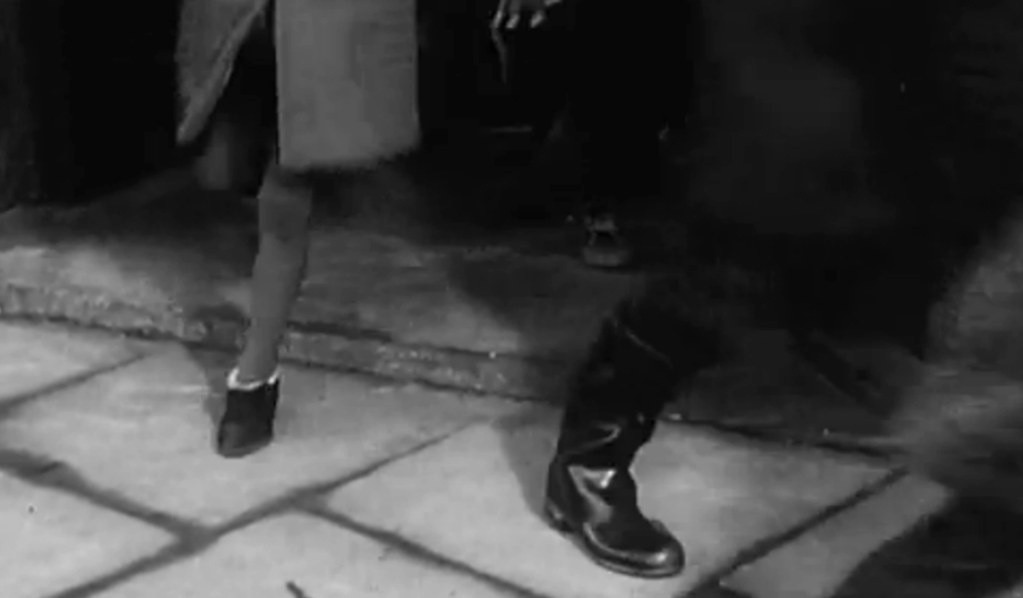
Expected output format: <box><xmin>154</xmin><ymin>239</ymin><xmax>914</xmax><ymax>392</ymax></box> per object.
<box><xmin>540</xmin><ymin>322</ymin><xmax>685</xmax><ymax>576</ymax></box>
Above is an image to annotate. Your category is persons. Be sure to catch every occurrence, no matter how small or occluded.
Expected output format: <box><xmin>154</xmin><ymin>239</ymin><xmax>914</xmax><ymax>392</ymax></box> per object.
<box><xmin>173</xmin><ymin>0</ymin><xmax>926</xmax><ymax>576</ymax></box>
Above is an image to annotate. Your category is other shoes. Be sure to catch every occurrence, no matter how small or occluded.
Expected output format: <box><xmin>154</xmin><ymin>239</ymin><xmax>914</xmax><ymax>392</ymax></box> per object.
<box><xmin>216</xmin><ymin>364</ymin><xmax>282</xmax><ymax>459</ymax></box>
<box><xmin>584</xmin><ymin>212</ymin><xmax>634</xmax><ymax>268</ymax></box>
